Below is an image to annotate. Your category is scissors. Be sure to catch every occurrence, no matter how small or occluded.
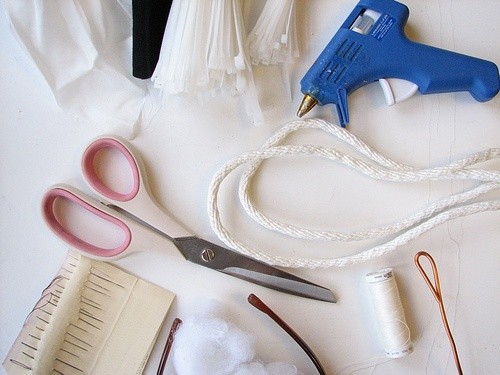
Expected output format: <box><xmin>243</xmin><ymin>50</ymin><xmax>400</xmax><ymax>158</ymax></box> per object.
<box><xmin>40</xmin><ymin>134</ymin><xmax>337</xmax><ymax>303</ymax></box>
